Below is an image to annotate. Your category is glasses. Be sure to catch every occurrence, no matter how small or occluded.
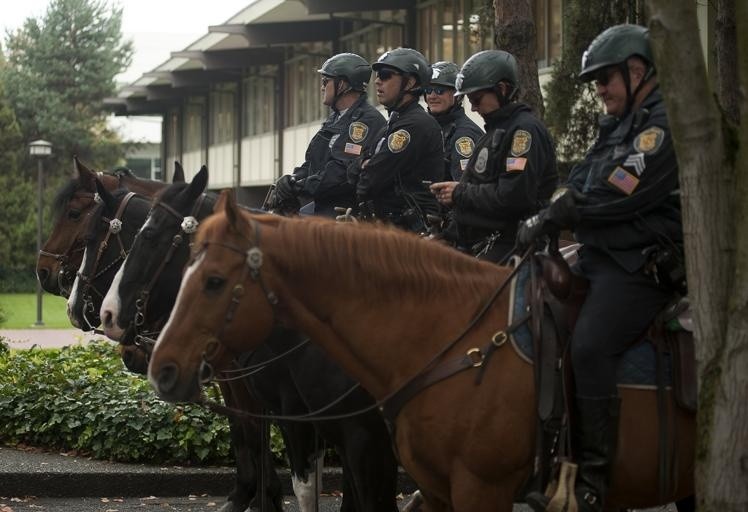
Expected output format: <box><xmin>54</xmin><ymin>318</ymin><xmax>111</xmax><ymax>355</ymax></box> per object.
<box><xmin>469</xmin><ymin>91</ymin><xmax>491</xmax><ymax>105</ymax></box>
<box><xmin>594</xmin><ymin>66</ymin><xmax>622</xmax><ymax>86</ymax></box>
<box><xmin>375</xmin><ymin>69</ymin><xmax>399</xmax><ymax>82</ymax></box>
<box><xmin>427</xmin><ymin>86</ymin><xmax>447</xmax><ymax>94</ymax></box>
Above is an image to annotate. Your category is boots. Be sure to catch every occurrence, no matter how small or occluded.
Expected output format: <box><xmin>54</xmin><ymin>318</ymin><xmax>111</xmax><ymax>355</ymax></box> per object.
<box><xmin>526</xmin><ymin>396</ymin><xmax>620</xmax><ymax>510</ymax></box>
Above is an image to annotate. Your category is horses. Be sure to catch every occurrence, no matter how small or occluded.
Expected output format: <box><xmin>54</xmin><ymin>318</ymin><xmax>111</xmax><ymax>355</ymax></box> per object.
<box><xmin>35</xmin><ymin>154</ymin><xmax>399</xmax><ymax>511</ymax></box>
<box><xmin>146</xmin><ymin>185</ymin><xmax>698</xmax><ymax>511</ymax></box>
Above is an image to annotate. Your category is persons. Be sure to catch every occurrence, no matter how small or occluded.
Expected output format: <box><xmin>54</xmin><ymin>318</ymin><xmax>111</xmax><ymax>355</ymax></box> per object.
<box><xmin>429</xmin><ymin>49</ymin><xmax>560</xmax><ymax>265</ymax></box>
<box><xmin>517</xmin><ymin>27</ymin><xmax>687</xmax><ymax>510</ymax></box>
<box><xmin>358</xmin><ymin>47</ymin><xmax>446</xmax><ymax>234</ymax></box>
<box><xmin>425</xmin><ymin>61</ymin><xmax>485</xmax><ymax>182</ymax></box>
<box><xmin>274</xmin><ymin>52</ymin><xmax>387</xmax><ymax>225</ymax></box>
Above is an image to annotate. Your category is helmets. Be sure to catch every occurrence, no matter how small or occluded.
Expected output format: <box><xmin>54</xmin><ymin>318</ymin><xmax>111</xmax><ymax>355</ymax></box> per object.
<box><xmin>317</xmin><ymin>52</ymin><xmax>373</xmax><ymax>87</ymax></box>
<box><xmin>373</xmin><ymin>46</ymin><xmax>431</xmax><ymax>92</ymax></box>
<box><xmin>577</xmin><ymin>24</ymin><xmax>655</xmax><ymax>80</ymax></box>
<box><xmin>428</xmin><ymin>60</ymin><xmax>460</xmax><ymax>86</ymax></box>
<box><xmin>454</xmin><ymin>49</ymin><xmax>519</xmax><ymax>95</ymax></box>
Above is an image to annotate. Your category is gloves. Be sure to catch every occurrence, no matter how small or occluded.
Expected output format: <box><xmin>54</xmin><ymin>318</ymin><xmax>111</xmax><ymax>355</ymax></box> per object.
<box><xmin>516</xmin><ymin>215</ymin><xmax>560</xmax><ymax>252</ymax></box>
<box><xmin>268</xmin><ymin>189</ymin><xmax>294</xmax><ymax>208</ymax></box>
<box><xmin>276</xmin><ymin>175</ymin><xmax>296</xmax><ymax>200</ymax></box>
<box><xmin>321</xmin><ymin>76</ymin><xmax>335</xmax><ymax>86</ymax></box>
